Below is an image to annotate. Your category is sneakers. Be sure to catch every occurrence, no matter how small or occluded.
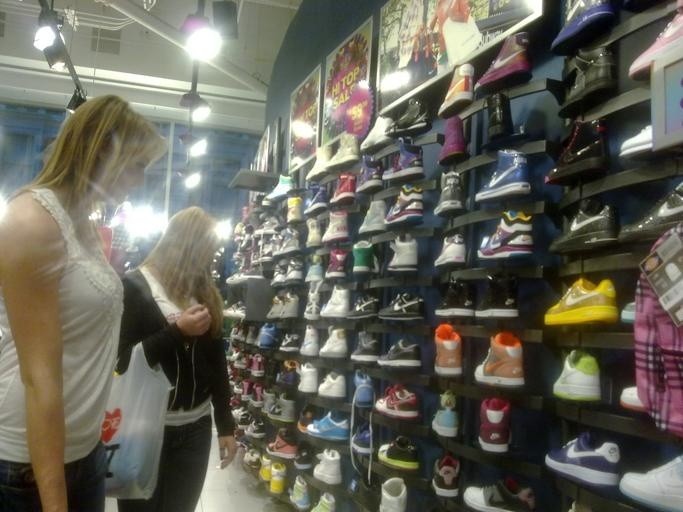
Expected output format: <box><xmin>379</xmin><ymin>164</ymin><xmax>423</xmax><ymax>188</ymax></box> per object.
<box><xmin>545</xmin><ymin>0</ymin><xmax>683</xmax><ymax>509</ymax></box>
<box><xmin>439</xmin><ymin>33</ymin><xmax>531</xmax><ymax>117</ymax></box>
<box><xmin>304</xmin><ymin>98</ymin><xmax>531</xmax><ymax>512</ymax></box>
<box><xmin>223</xmin><ymin>173</ymin><xmax>310</xmax><ymax>509</ymax></box>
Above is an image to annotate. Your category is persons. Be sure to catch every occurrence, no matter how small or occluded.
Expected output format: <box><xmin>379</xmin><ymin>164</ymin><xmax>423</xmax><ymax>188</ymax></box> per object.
<box><xmin>115</xmin><ymin>206</ymin><xmax>237</xmax><ymax>512</ymax></box>
<box><xmin>0</xmin><ymin>94</ymin><xmax>169</xmax><ymax>512</ymax></box>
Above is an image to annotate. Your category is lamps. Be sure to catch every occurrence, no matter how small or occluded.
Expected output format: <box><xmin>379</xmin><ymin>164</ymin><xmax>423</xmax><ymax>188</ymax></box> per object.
<box><xmin>32</xmin><ymin>1</ymin><xmax>224</xmax><ymax>191</ymax></box>
